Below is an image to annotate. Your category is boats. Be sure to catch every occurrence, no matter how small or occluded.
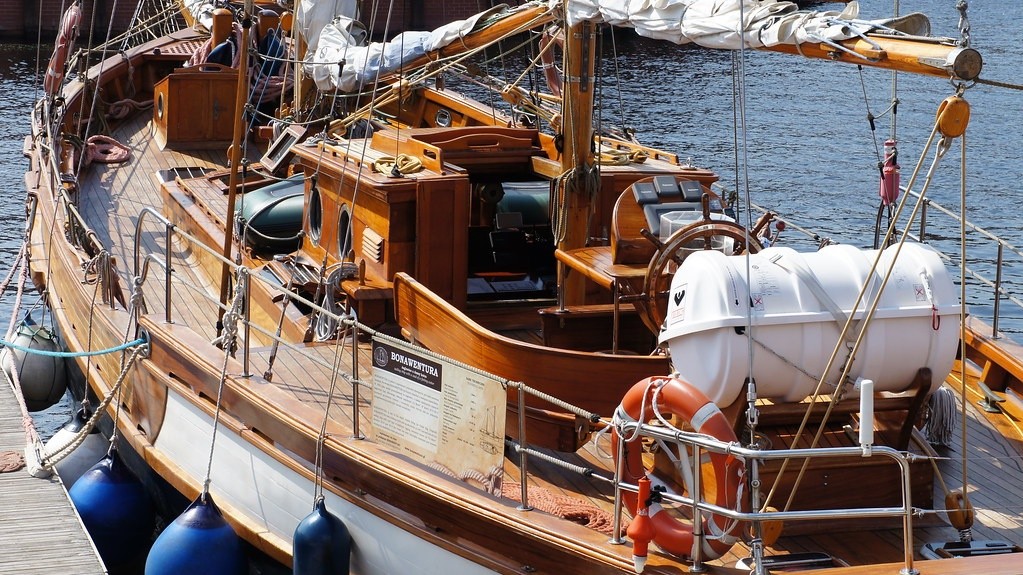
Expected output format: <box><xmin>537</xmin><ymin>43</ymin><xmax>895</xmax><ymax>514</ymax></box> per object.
<box><xmin>233</xmin><ymin>171</ymin><xmax>550</xmax><ymax>273</ymax></box>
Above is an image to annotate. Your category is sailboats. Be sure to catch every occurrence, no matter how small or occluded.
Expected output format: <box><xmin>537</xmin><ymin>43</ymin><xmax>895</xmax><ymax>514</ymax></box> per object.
<box><xmin>22</xmin><ymin>0</ymin><xmax>1023</xmax><ymax>575</ymax></box>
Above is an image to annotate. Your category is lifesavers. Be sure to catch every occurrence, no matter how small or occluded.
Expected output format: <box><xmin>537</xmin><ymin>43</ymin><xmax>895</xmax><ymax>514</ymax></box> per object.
<box><xmin>610</xmin><ymin>375</ymin><xmax>748</xmax><ymax>563</ymax></box>
<box><xmin>540</xmin><ymin>26</ymin><xmax>566</xmax><ymax>97</ymax></box>
<box><xmin>44</xmin><ymin>4</ymin><xmax>81</xmax><ymax>92</ymax></box>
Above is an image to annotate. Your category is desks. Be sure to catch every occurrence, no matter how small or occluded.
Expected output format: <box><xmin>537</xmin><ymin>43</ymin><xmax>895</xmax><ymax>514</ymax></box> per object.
<box><xmin>554</xmin><ymin>246</ymin><xmax>677</xmax><ymax>352</ymax></box>
